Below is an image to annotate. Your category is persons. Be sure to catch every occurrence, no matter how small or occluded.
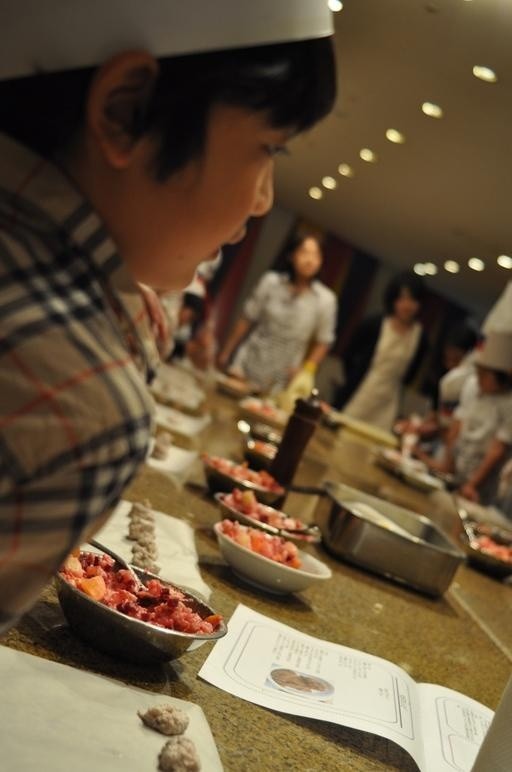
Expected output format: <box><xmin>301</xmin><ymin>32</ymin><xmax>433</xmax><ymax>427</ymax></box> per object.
<box><xmin>339</xmin><ymin>268</ymin><xmax>430</xmax><ymax>433</ymax></box>
<box><xmin>168</xmin><ymin>279</ymin><xmax>214</xmax><ymax>354</ymax></box>
<box><xmin>218</xmin><ymin>225</ymin><xmax>336</xmax><ymax>403</ymax></box>
<box><xmin>395</xmin><ymin>399</ymin><xmax>459</xmax><ymax>473</ymax></box>
<box><xmin>1</xmin><ymin>4</ymin><xmax>340</xmax><ymax>637</ymax></box>
<box><xmin>412</xmin><ymin>285</ymin><xmax>512</xmax><ymax>505</ymax></box>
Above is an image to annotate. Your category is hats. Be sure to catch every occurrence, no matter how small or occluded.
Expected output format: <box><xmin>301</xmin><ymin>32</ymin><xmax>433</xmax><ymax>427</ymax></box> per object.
<box><xmin>472</xmin><ymin>281</ymin><xmax>512</xmax><ymax>374</ymax></box>
<box><xmin>0</xmin><ymin>0</ymin><xmax>338</xmax><ymax>82</ymax></box>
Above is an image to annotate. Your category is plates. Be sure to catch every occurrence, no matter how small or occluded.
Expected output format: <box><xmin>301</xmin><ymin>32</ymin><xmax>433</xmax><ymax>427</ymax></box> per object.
<box><xmin>401</xmin><ymin>468</ymin><xmax>443</xmax><ymax>490</ymax></box>
<box><xmin>237</xmin><ymin>394</ymin><xmax>288</xmax><ymax>429</ymax></box>
<box><xmin>242</xmin><ymin>438</ymin><xmax>278</xmax><ymax>463</ymax></box>
<box><xmin>209</xmin><ymin>372</ymin><xmax>259</xmax><ymax>398</ymax></box>
<box><xmin>465</xmin><ymin>539</ymin><xmax>511</xmax><ymax>583</ymax></box>
<box><xmin>249</xmin><ymin>421</ymin><xmax>279</xmax><ymax>445</ymax></box>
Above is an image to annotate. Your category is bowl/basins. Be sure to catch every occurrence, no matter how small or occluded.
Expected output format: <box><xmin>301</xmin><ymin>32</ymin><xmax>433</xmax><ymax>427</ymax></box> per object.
<box><xmin>212</xmin><ymin>521</ymin><xmax>332</xmax><ymax>596</ymax></box>
<box><xmin>50</xmin><ymin>552</ymin><xmax>225</xmax><ymax>668</ymax></box>
<box><xmin>313</xmin><ymin>478</ymin><xmax>464</xmax><ymax>601</ymax></box>
<box><xmin>199</xmin><ymin>455</ymin><xmax>284</xmax><ymax>504</ymax></box>
<box><xmin>213</xmin><ymin>492</ymin><xmax>322</xmax><ymax>549</ymax></box>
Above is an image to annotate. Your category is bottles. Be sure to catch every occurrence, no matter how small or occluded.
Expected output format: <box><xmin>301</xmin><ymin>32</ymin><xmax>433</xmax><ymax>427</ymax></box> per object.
<box><xmin>268</xmin><ymin>387</ymin><xmax>324</xmax><ymax>489</ymax></box>
